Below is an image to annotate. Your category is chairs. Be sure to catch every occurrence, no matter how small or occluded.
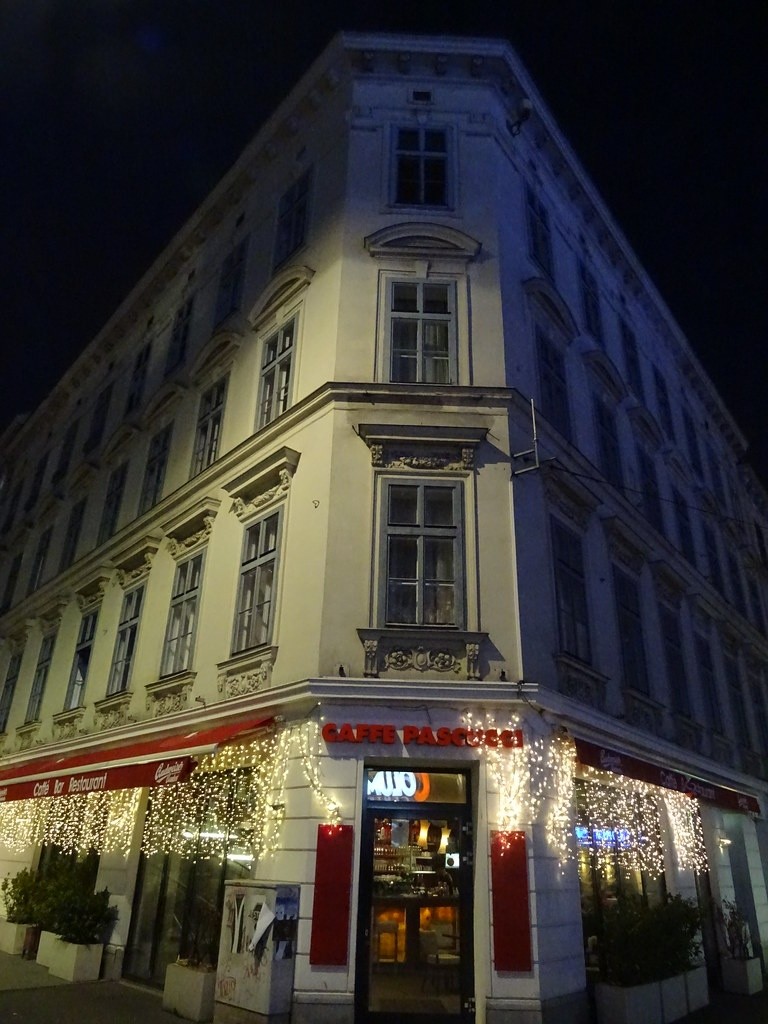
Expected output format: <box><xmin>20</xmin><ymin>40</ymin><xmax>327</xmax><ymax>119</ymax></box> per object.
<box><xmin>418</xmin><ymin>928</ymin><xmax>461</xmax><ymax>995</ymax></box>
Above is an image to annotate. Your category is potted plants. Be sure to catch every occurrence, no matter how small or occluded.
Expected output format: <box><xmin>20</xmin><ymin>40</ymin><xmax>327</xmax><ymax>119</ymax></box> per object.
<box><xmin>715</xmin><ymin>896</ymin><xmax>764</xmax><ymax>995</ymax></box>
<box><xmin>593</xmin><ymin>892</ymin><xmax>710</xmax><ymax>1024</ymax></box>
<box><xmin>161</xmin><ymin>898</ymin><xmax>220</xmax><ymax>1024</ymax></box>
<box><xmin>0</xmin><ymin>866</ymin><xmax>43</xmax><ymax>954</ymax></box>
<box><xmin>49</xmin><ymin>881</ymin><xmax>120</xmax><ymax>982</ymax></box>
<box><xmin>29</xmin><ymin>872</ymin><xmax>72</xmax><ymax>968</ymax></box>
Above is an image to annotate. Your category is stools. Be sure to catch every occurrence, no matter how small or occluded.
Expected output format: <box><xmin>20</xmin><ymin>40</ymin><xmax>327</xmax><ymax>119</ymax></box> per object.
<box><xmin>375</xmin><ymin>921</ymin><xmax>399</xmax><ymax>975</ymax></box>
